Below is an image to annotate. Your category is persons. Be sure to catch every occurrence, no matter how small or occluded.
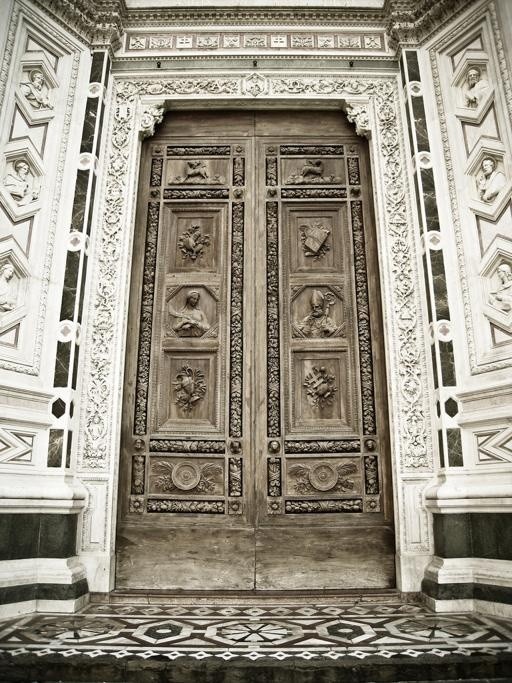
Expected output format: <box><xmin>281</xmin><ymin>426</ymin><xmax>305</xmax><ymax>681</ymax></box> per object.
<box><xmin>465</xmin><ymin>68</ymin><xmax>490</xmax><ymax>111</ymax></box>
<box><xmin>492</xmin><ymin>261</ymin><xmax>512</xmax><ymax>312</ymax></box>
<box><xmin>294</xmin><ymin>288</ymin><xmax>345</xmax><ymax>338</ymax></box>
<box><xmin>475</xmin><ymin>153</ymin><xmax>509</xmax><ymax>201</ymax></box>
<box><xmin>23</xmin><ymin>69</ymin><xmax>51</xmax><ymax>109</ymax></box>
<box><xmin>172</xmin><ymin>293</ymin><xmax>211</xmax><ymax>336</ymax></box>
<box><xmin>0</xmin><ymin>263</ymin><xmax>17</xmax><ymax>313</ymax></box>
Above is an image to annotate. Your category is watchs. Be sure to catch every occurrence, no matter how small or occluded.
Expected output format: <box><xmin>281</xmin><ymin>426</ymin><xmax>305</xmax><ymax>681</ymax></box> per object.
<box><xmin>7</xmin><ymin>159</ymin><xmax>41</xmax><ymax>207</ymax></box>
